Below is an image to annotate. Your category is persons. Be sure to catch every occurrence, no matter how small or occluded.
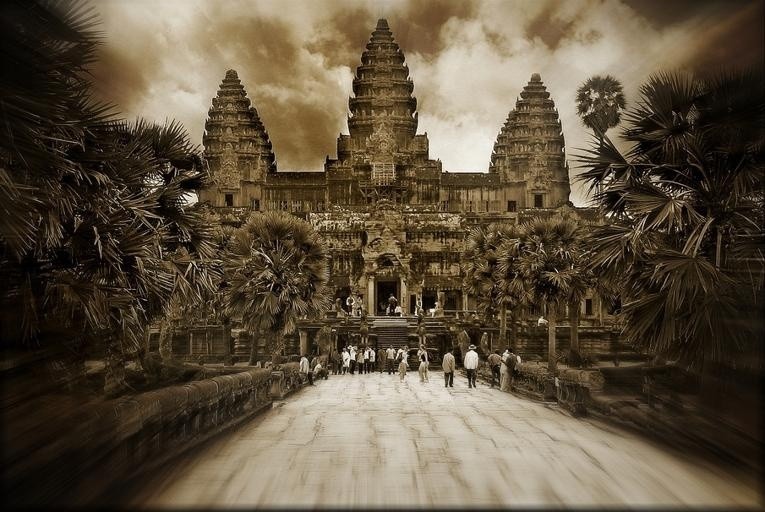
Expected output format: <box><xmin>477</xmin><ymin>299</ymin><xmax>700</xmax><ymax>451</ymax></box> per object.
<box><xmin>300</xmin><ymin>293</ymin><xmax>522</xmax><ymax>391</ymax></box>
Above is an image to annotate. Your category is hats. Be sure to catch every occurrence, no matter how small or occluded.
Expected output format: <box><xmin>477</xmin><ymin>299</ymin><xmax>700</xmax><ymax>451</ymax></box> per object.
<box><xmin>469</xmin><ymin>345</ymin><xmax>477</xmax><ymax>350</ymax></box>
